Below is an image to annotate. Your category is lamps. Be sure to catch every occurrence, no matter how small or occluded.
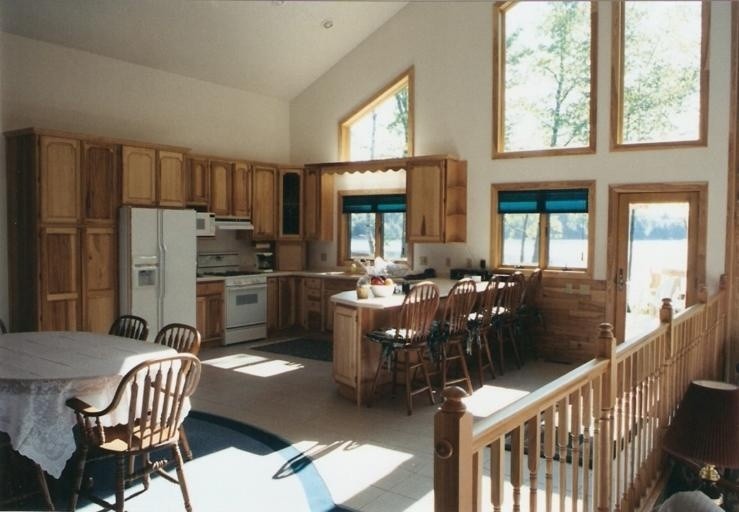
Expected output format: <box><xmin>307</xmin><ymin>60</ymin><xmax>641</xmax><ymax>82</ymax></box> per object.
<box><xmin>662</xmin><ymin>380</ymin><xmax>738</xmax><ymax>508</ymax></box>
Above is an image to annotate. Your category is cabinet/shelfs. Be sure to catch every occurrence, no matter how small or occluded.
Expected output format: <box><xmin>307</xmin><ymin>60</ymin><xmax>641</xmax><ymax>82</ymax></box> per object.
<box><xmin>4</xmin><ymin>126</ymin><xmax>118</xmax><ymax>225</ymax></box>
<box><xmin>406</xmin><ymin>153</ymin><xmax>466</xmax><ymax>244</ymax></box>
<box><xmin>118</xmin><ymin>138</ymin><xmax>191</xmax><ymax>209</ymax></box>
<box><xmin>189</xmin><ymin>154</ymin><xmax>333</xmax><ymax>244</ymax></box>
<box><xmin>267</xmin><ymin>274</ymin><xmax>358</xmax><ymax>339</ymax></box>
<box><xmin>198</xmin><ymin>276</ymin><xmax>223</xmax><ymax>345</ymax></box>
<box><xmin>9</xmin><ymin>224</ymin><xmax>119</xmax><ymax>334</ymax></box>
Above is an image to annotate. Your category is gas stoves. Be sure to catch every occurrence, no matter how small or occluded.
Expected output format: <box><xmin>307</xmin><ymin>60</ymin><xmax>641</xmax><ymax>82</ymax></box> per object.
<box><xmin>203</xmin><ymin>269</ymin><xmax>267</xmax><ymax>286</ymax></box>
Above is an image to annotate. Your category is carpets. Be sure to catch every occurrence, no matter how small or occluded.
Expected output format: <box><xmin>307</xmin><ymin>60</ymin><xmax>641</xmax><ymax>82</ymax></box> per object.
<box><xmin>484</xmin><ymin>424</ymin><xmax>620</xmax><ymax>469</ymax></box>
<box><xmin>250</xmin><ymin>337</ymin><xmax>333</xmax><ymax>362</ymax></box>
<box><xmin>44</xmin><ymin>411</ymin><xmax>353</xmax><ymax>511</ymax></box>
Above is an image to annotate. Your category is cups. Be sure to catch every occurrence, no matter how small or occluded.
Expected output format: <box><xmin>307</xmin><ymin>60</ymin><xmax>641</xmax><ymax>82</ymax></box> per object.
<box><xmin>471</xmin><ymin>275</ymin><xmax>481</xmax><ymax>283</ymax></box>
<box><xmin>351</xmin><ymin>263</ymin><xmax>356</xmax><ymax>273</ymax></box>
<box><xmin>356</xmin><ymin>286</ymin><xmax>369</xmax><ymax>299</ymax></box>
<box><xmin>402</xmin><ymin>282</ymin><xmax>410</xmax><ymax>293</ymax></box>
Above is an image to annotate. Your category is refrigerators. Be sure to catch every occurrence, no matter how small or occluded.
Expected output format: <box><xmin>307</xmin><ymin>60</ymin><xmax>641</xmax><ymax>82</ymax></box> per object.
<box><xmin>118</xmin><ymin>206</ymin><xmax>197</xmax><ymax>343</ymax></box>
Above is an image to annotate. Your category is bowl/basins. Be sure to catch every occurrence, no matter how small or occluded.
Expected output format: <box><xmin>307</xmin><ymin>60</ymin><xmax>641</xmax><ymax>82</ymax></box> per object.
<box><xmin>371</xmin><ymin>284</ymin><xmax>396</xmax><ymax>297</ymax></box>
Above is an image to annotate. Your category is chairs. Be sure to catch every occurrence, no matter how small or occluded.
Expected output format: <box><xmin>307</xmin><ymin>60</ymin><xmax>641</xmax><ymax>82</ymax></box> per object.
<box><xmin>1</xmin><ymin>316</ymin><xmax>201</xmax><ymax>511</ymax></box>
<box><xmin>366</xmin><ymin>266</ymin><xmax>543</xmax><ymax>415</ymax></box>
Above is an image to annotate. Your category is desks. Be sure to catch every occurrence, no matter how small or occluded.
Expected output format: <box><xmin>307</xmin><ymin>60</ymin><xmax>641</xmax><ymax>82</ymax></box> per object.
<box><xmin>327</xmin><ymin>277</ymin><xmax>522</xmax><ymax>404</ymax></box>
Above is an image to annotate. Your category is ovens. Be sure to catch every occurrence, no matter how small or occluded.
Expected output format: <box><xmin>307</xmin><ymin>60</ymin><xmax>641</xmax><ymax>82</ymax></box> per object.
<box><xmin>221</xmin><ymin>283</ymin><xmax>268</xmax><ymax>346</ymax></box>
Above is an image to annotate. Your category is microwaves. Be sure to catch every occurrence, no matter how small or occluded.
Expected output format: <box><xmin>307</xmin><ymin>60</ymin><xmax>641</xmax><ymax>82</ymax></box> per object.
<box><xmin>197</xmin><ymin>212</ymin><xmax>216</xmax><ymax>236</ymax></box>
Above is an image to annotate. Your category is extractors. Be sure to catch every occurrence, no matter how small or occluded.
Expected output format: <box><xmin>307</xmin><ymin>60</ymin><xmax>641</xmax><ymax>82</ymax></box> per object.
<box><xmin>216</xmin><ymin>220</ymin><xmax>255</xmax><ymax>232</ymax></box>
